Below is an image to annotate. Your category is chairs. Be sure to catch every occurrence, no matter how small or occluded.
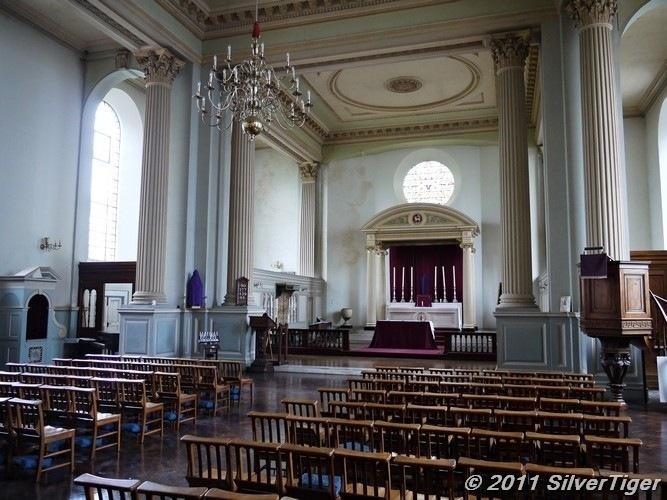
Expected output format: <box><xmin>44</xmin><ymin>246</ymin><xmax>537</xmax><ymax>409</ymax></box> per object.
<box><xmin>0</xmin><ymin>353</ymin><xmax>667</xmax><ymax>500</ymax></box>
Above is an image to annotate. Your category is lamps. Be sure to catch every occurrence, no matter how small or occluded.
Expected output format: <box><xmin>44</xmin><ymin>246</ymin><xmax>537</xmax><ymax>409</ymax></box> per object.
<box><xmin>192</xmin><ymin>1</ymin><xmax>313</xmax><ymax>144</ymax></box>
<box><xmin>40</xmin><ymin>235</ymin><xmax>63</xmax><ymax>253</ymax></box>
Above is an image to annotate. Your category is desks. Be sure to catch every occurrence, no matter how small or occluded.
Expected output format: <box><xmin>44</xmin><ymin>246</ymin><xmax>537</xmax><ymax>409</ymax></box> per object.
<box><xmin>202</xmin><ymin>344</ymin><xmax>219</xmax><ymax>362</ymax></box>
